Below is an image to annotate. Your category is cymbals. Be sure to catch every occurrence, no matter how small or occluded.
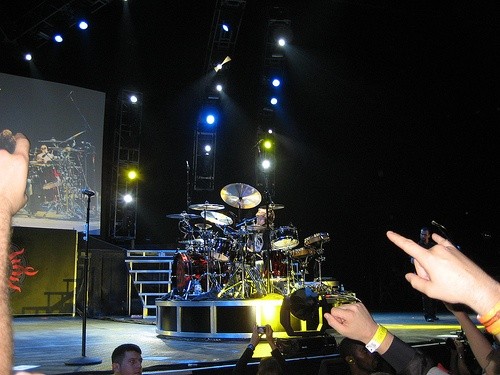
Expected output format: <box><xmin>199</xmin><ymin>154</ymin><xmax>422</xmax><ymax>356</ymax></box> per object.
<box><xmin>219</xmin><ymin>182</ymin><xmax>262</xmax><ymax>210</ymax></box>
<box><xmin>37</xmin><ymin>140</ymin><xmax>67</xmax><ymax>143</ymax></box>
<box><xmin>255</xmin><ymin>204</ymin><xmax>285</xmax><ymax>210</ymax></box>
<box><xmin>194</xmin><ymin>223</ymin><xmax>213</xmax><ymax>230</ymax></box>
<box><xmin>165</xmin><ymin>214</ymin><xmax>202</xmax><ymax>219</ymax></box>
<box><xmin>59</xmin><ymin>129</ymin><xmax>87</xmax><ymax>146</ymax></box>
<box><xmin>188</xmin><ymin>203</ymin><xmax>225</xmax><ymax>211</ymax></box>
<box><xmin>28</xmin><ymin>160</ymin><xmax>52</xmax><ymax>167</ymax></box>
<box><xmin>200</xmin><ymin>211</ymin><xmax>234</xmax><ymax>226</ymax></box>
<box><xmin>54</xmin><ymin>147</ymin><xmax>86</xmax><ymax>153</ymax></box>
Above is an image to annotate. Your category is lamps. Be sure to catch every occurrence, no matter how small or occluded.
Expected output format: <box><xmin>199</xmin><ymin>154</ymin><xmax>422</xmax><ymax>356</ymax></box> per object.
<box><xmin>280</xmin><ymin>288</ymin><xmax>332</xmax><ymax>336</ymax></box>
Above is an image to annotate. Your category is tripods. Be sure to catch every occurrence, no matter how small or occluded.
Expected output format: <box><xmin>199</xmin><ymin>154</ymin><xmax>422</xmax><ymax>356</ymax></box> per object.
<box><xmin>178</xmin><ymin>211</ymin><xmax>334</xmax><ymax>299</ymax></box>
<box><xmin>45</xmin><ymin>145</ymin><xmax>99</xmax><ymax>220</ymax></box>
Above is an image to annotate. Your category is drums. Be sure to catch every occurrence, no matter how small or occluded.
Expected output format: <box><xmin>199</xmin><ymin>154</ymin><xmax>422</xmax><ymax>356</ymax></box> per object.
<box><xmin>177</xmin><ymin>238</ymin><xmax>209</xmax><ymax>254</ymax></box>
<box><xmin>172</xmin><ymin>251</ymin><xmax>230</xmax><ymax>295</ymax></box>
<box><xmin>259</xmin><ymin>249</ymin><xmax>292</xmax><ymax>282</ymax></box>
<box><xmin>289</xmin><ymin>247</ymin><xmax>316</xmax><ymax>260</ymax></box>
<box><xmin>37</xmin><ymin>164</ymin><xmax>63</xmax><ymax>191</ymax></box>
<box><xmin>237</xmin><ymin>225</ymin><xmax>272</xmax><ymax>260</ymax></box>
<box><xmin>303</xmin><ymin>232</ymin><xmax>331</xmax><ymax>248</ymax></box>
<box><xmin>270</xmin><ymin>226</ymin><xmax>300</xmax><ymax>249</ymax></box>
<box><xmin>198</xmin><ymin>232</ymin><xmax>219</xmax><ymax>251</ymax></box>
<box><xmin>210</xmin><ymin>237</ymin><xmax>235</xmax><ymax>263</ymax></box>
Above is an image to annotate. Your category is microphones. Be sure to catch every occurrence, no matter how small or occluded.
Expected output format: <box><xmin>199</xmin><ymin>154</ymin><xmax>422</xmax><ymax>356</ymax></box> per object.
<box><xmin>81</xmin><ymin>189</ymin><xmax>95</xmax><ymax>196</ymax></box>
<box><xmin>431</xmin><ymin>221</ymin><xmax>447</xmax><ymax>229</ymax></box>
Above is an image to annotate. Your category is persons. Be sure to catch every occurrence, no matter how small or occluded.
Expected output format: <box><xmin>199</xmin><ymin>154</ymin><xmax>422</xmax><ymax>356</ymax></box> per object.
<box><xmin>0</xmin><ymin>133</ymin><xmax>31</xmax><ymax>375</ymax></box>
<box><xmin>442</xmin><ymin>301</ymin><xmax>500</xmax><ymax>375</ymax></box>
<box><xmin>386</xmin><ymin>231</ymin><xmax>500</xmax><ymax>343</ymax></box>
<box><xmin>236</xmin><ymin>324</ymin><xmax>288</xmax><ymax>375</ymax></box>
<box><xmin>411</xmin><ymin>228</ymin><xmax>439</xmax><ymax>322</ymax></box>
<box><xmin>243</xmin><ymin>208</ymin><xmax>275</xmax><ymax>252</ymax></box>
<box><xmin>338</xmin><ymin>337</ymin><xmax>378</xmax><ymax>375</ymax></box>
<box><xmin>37</xmin><ymin>144</ymin><xmax>55</xmax><ymax>162</ymax></box>
<box><xmin>324</xmin><ymin>303</ymin><xmax>451</xmax><ymax>375</ymax></box>
<box><xmin>450</xmin><ymin>341</ymin><xmax>471</xmax><ymax>375</ymax></box>
<box><xmin>111</xmin><ymin>343</ymin><xmax>143</xmax><ymax>375</ymax></box>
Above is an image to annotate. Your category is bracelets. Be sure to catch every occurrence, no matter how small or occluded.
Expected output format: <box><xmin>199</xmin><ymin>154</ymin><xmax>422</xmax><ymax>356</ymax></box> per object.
<box><xmin>365</xmin><ymin>324</ymin><xmax>390</xmax><ymax>354</ymax></box>
<box><xmin>477</xmin><ymin>301</ymin><xmax>500</xmax><ymax>336</ymax></box>
<box><xmin>456</xmin><ymin>352</ymin><xmax>465</xmax><ymax>359</ymax></box>
<box><xmin>247</xmin><ymin>344</ymin><xmax>255</xmax><ymax>350</ymax></box>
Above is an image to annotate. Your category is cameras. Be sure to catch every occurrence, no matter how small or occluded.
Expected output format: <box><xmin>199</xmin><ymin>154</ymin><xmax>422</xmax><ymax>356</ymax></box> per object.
<box><xmin>257</xmin><ymin>327</ymin><xmax>266</xmax><ymax>334</ymax></box>
